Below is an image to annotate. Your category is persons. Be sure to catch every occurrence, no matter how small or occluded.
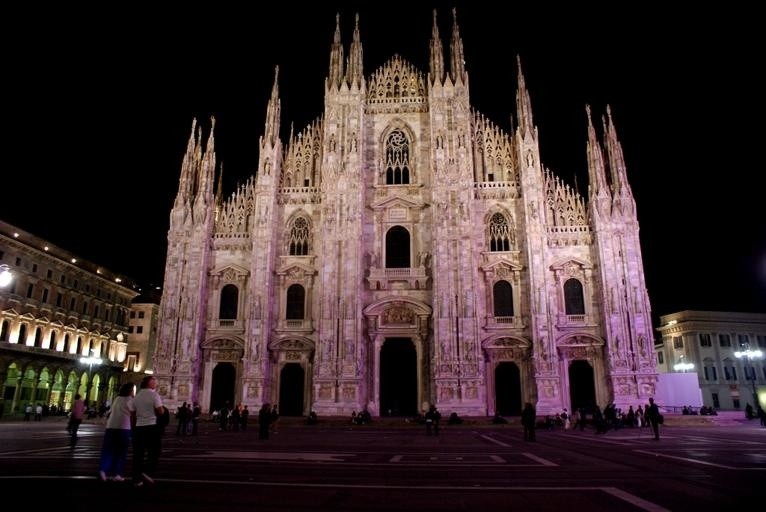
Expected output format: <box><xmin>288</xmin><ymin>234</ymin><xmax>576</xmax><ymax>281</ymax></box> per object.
<box><xmin>682</xmin><ymin>405</ymin><xmax>718</xmax><ymax>416</ymax></box>
<box><xmin>521</xmin><ymin>398</ymin><xmax>661</xmax><ymax>442</ymax></box>
<box><xmin>745</xmin><ymin>403</ymin><xmax>753</xmax><ymax>420</ymax></box>
<box><xmin>413</xmin><ymin>405</ymin><xmax>440</xmax><ymax>436</ymax></box>
<box><xmin>303</xmin><ymin>411</ymin><xmax>318</xmax><ymax>426</ymax></box>
<box><xmin>349</xmin><ymin>409</ymin><xmax>372</xmax><ymax>426</ymax></box>
<box><xmin>24</xmin><ymin>376</ymin><xmax>281</xmax><ymax>486</ymax></box>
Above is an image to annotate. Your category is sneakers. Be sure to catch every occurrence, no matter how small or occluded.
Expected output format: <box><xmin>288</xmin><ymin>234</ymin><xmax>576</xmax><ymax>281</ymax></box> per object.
<box><xmin>97</xmin><ymin>470</ymin><xmax>126</xmax><ymax>483</ymax></box>
<box><xmin>134</xmin><ymin>473</ymin><xmax>156</xmax><ymax>487</ymax></box>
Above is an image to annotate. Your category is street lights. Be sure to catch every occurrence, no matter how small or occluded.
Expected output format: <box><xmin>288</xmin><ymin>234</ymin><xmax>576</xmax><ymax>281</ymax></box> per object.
<box><xmin>674</xmin><ymin>353</ymin><xmax>694</xmax><ymax>373</ymax></box>
<box><xmin>735</xmin><ymin>341</ymin><xmax>766</xmax><ymax>426</ymax></box>
<box><xmin>77</xmin><ymin>349</ymin><xmax>104</xmax><ymax>410</ymax></box>
<box><xmin>0</xmin><ymin>263</ymin><xmax>12</xmax><ymax>287</ymax></box>
